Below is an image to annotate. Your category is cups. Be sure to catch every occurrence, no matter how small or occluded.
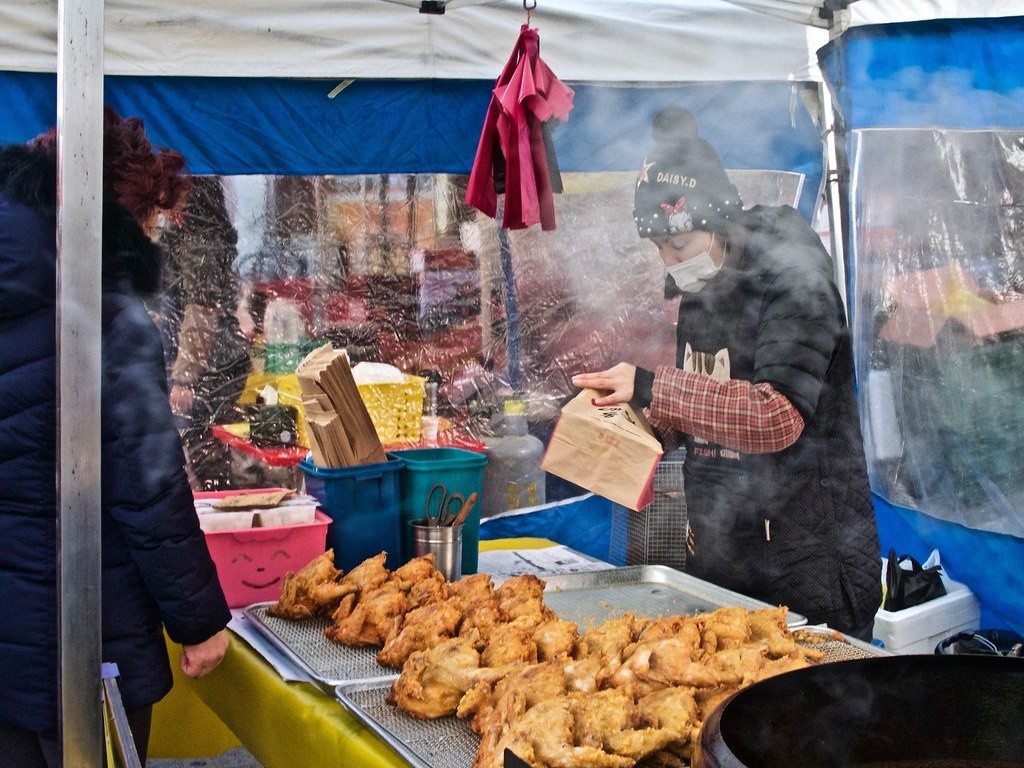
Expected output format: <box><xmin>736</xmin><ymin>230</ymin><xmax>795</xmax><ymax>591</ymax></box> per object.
<box><xmin>409</xmin><ymin>518</ymin><xmax>462</xmax><ymax>583</ymax></box>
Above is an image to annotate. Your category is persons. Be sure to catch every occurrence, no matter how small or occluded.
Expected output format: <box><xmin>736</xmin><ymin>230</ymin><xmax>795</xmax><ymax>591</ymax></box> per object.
<box><xmin>571</xmin><ymin>106</ymin><xmax>882</xmax><ymax>646</ymax></box>
<box><xmin>1</xmin><ymin>111</ymin><xmax>254</xmax><ymax>768</ymax></box>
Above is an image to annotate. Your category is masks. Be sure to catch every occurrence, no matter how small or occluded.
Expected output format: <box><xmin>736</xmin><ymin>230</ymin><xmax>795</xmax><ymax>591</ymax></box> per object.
<box><xmin>666</xmin><ymin>231</ymin><xmax>728</xmax><ymax>292</ymax></box>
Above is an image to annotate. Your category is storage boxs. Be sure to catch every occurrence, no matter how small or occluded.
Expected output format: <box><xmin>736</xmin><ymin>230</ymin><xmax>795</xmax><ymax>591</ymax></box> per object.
<box><xmin>190</xmin><ymin>488</ymin><xmax>333</xmax><ymax>612</ymax></box>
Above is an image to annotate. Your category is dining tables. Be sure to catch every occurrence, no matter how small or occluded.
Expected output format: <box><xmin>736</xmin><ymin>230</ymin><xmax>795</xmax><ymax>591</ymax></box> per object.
<box><xmin>164</xmin><ymin>538</ymin><xmax>900</xmax><ymax>768</ymax></box>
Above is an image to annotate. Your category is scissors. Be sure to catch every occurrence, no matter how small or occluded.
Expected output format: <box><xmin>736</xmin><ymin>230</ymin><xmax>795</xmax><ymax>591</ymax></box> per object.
<box><xmin>423</xmin><ymin>484</ymin><xmax>466</xmax><ymax>528</ymax></box>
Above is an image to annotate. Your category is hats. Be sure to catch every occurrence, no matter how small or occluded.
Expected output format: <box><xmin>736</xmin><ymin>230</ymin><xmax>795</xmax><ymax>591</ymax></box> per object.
<box><xmin>632</xmin><ymin>106</ymin><xmax>744</xmax><ymax>237</ymax></box>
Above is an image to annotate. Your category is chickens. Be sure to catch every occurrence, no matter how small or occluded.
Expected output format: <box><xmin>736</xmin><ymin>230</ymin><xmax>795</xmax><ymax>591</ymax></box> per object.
<box><xmin>262</xmin><ymin>549</ymin><xmax>851</xmax><ymax>768</ymax></box>
